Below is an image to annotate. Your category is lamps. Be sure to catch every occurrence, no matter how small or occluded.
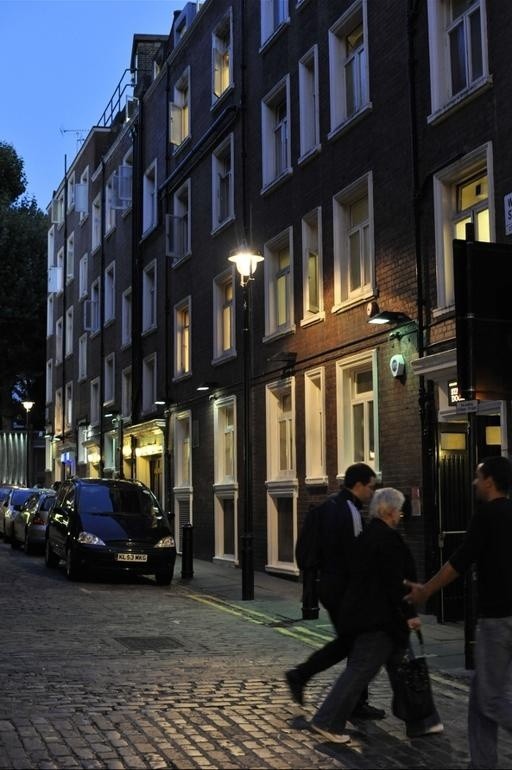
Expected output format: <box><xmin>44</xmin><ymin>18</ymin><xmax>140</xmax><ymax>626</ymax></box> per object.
<box><xmin>45</xmin><ymin>433</ymin><xmax>53</xmax><ymax>438</ymax></box>
<box><xmin>78</xmin><ymin>422</ymin><xmax>90</xmax><ymax>427</ymax></box>
<box><xmin>196</xmin><ymin>382</ymin><xmax>217</xmax><ymax>391</ymax></box>
<box><xmin>54</xmin><ymin>435</ymin><xmax>64</xmax><ymax>440</ymax></box>
<box><xmin>104</xmin><ymin>410</ymin><xmax>119</xmax><ymax>417</ymax></box>
<box><xmin>367</xmin><ymin>311</ymin><xmax>397</xmax><ymax>325</ymax></box>
<box><xmin>154</xmin><ymin>398</ymin><xmax>172</xmax><ymax>405</ymax></box>
<box><xmin>266</xmin><ymin>352</ymin><xmax>297</xmax><ymax>362</ymax></box>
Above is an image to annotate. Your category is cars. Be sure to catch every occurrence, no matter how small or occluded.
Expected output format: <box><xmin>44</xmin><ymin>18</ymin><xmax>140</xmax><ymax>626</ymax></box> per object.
<box><xmin>0</xmin><ymin>486</ymin><xmax>56</xmax><ymax>557</ymax></box>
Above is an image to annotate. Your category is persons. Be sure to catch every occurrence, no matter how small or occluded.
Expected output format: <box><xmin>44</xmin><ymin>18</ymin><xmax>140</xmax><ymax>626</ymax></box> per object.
<box><xmin>401</xmin><ymin>455</ymin><xmax>512</xmax><ymax>769</ymax></box>
<box><xmin>33</xmin><ymin>483</ymin><xmax>43</xmax><ymax>489</ymax></box>
<box><xmin>50</xmin><ymin>480</ymin><xmax>62</xmax><ymax>491</ymax></box>
<box><xmin>309</xmin><ymin>488</ymin><xmax>445</xmax><ymax>745</ymax></box>
<box><xmin>282</xmin><ymin>463</ymin><xmax>386</xmax><ymax>720</ymax></box>
<box><xmin>71</xmin><ymin>475</ymin><xmax>79</xmax><ymax>479</ymax></box>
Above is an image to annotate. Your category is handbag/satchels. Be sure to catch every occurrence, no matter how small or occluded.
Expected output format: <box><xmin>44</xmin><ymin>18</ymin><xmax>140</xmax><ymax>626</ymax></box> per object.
<box><xmin>390</xmin><ymin>659</ymin><xmax>433</xmax><ymax>721</ymax></box>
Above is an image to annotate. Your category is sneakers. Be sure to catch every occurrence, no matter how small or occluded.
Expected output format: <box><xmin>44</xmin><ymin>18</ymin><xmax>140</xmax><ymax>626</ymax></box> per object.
<box><xmin>423</xmin><ymin>725</ymin><xmax>443</xmax><ymax>736</ymax></box>
<box><xmin>309</xmin><ymin>724</ymin><xmax>351</xmax><ymax>744</ymax></box>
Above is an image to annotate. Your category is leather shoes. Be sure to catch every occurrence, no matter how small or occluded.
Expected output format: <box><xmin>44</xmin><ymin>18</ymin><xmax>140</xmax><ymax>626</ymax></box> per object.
<box><xmin>286</xmin><ymin>668</ymin><xmax>304</xmax><ymax>706</ymax></box>
<box><xmin>351</xmin><ymin>703</ymin><xmax>386</xmax><ymax>720</ymax></box>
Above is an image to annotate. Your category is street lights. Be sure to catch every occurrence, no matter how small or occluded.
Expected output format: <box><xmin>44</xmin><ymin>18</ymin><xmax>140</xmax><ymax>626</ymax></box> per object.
<box><xmin>227</xmin><ymin>244</ymin><xmax>267</xmax><ymax>603</ymax></box>
<box><xmin>18</xmin><ymin>399</ymin><xmax>37</xmax><ymax>488</ymax></box>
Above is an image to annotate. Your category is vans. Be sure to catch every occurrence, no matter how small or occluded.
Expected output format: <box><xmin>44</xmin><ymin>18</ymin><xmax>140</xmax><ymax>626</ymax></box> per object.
<box><xmin>42</xmin><ymin>475</ymin><xmax>178</xmax><ymax>587</ymax></box>
